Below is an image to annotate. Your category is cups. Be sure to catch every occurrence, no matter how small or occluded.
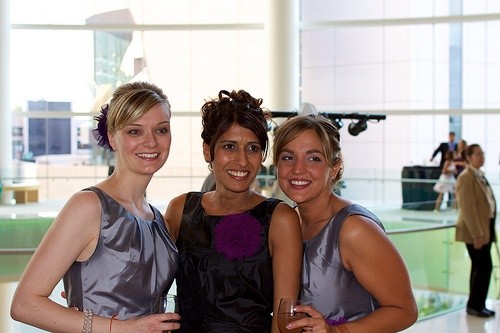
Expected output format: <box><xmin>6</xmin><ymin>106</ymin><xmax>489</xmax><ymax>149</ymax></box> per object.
<box><xmin>276</xmin><ymin>297</ymin><xmax>306</xmax><ymax>333</ymax></box>
<box><xmin>149</xmin><ymin>293</ymin><xmax>180</xmax><ymax>333</ymax></box>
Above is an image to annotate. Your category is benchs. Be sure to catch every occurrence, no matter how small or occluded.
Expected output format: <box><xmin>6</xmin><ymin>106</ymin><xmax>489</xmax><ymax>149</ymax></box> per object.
<box><xmin>3</xmin><ymin>187</ymin><xmax>39</xmax><ymax>204</ymax></box>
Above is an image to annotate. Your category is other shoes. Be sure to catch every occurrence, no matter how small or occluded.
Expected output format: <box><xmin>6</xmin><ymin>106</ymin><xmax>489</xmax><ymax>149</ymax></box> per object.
<box><xmin>466</xmin><ymin>307</ymin><xmax>495</xmax><ymax>316</ymax></box>
